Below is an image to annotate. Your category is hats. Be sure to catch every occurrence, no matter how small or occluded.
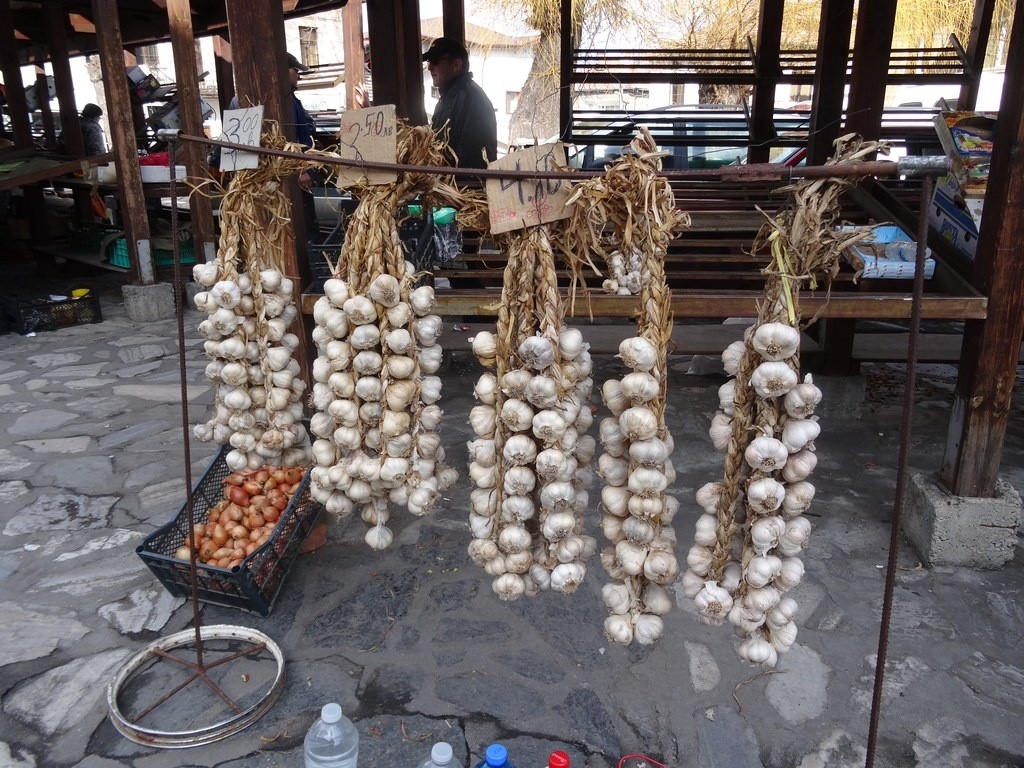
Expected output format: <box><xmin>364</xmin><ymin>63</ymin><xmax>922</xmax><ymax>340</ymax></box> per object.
<box><xmin>287</xmin><ymin>52</ymin><xmax>310</xmax><ymax>72</ymax></box>
<box><xmin>83</xmin><ymin>104</ymin><xmax>103</xmax><ymax>118</ymax></box>
<box><xmin>423</xmin><ymin>37</ymin><xmax>469</xmax><ymax>62</ymax></box>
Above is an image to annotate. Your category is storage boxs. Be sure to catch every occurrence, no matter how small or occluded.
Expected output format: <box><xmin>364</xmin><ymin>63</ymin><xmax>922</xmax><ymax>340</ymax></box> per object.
<box><xmin>930</xmin><ymin>177</ymin><xmax>980</xmax><ymax>267</ymax></box>
<box><xmin>108</xmin><ymin>237</ymin><xmax>197</xmax><ymax>269</ymax></box>
<box><xmin>129</xmin><ymin>74</ymin><xmax>161</xmax><ymax>106</ymax></box>
<box><xmin>143</xmin><ymin>92</ymin><xmax>214</xmax><ymax>133</ymax></box>
<box><xmin>6</xmin><ymin>215</ymin><xmax>67</xmax><ymax>240</ymax></box>
<box><xmin>306</xmin><ymin>214</ymin><xmax>437</xmax><ymax>293</ymax></box>
<box><xmin>0</xmin><ymin>279</ymin><xmax>103</xmax><ymax>336</ymax></box>
<box><xmin>835</xmin><ymin>222</ymin><xmax>936</xmax><ymax>281</ymax></box>
<box><xmin>76</xmin><ymin>220</ymin><xmax>125</xmax><ymax>254</ymax></box>
<box><xmin>931</xmin><ymin>110</ymin><xmax>1001</xmax><ymax>161</ymax></box>
<box><xmin>104</xmin><ymin>193</ymin><xmax>162</xmax><ymax>227</ymax></box>
<box><xmin>138</xmin><ymin>165</ymin><xmax>187</xmax><ymax>183</ymax></box>
<box><xmin>137</xmin><ymin>438</ymin><xmax>327</xmax><ymax>616</ymax></box>
<box><xmin>126</xmin><ymin>64</ymin><xmax>145</xmax><ymax>84</ymax></box>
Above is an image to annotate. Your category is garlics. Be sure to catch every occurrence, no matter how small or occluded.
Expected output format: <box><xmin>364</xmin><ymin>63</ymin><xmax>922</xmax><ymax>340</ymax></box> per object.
<box><xmin>190</xmin><ymin>264</ymin><xmax>469</xmax><ymax>553</ymax></box>
<box><xmin>466</xmin><ymin>245</ymin><xmax>825</xmax><ymax>673</ymax></box>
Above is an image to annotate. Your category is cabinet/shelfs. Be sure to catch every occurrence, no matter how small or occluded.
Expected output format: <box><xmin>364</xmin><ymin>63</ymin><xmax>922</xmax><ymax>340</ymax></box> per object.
<box><xmin>559</xmin><ymin>0</ymin><xmax>996</xmax><ymax>171</ymax></box>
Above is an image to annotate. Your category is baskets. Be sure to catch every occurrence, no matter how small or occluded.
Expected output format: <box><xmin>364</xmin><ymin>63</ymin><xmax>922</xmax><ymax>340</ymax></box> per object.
<box><xmin>109</xmin><ymin>228</ymin><xmax>197</xmax><ymax>268</ymax></box>
<box><xmin>135</xmin><ymin>415</ymin><xmax>335</xmax><ymax>618</ymax></box>
<box><xmin>304</xmin><ymin>205</ymin><xmax>438</xmax><ymax>298</ymax></box>
<box><xmin>11</xmin><ymin>286</ymin><xmax>104</xmax><ymax>335</ymax></box>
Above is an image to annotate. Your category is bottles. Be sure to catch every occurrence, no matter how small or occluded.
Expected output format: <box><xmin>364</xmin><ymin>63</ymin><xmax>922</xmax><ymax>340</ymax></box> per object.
<box><xmin>416</xmin><ymin>742</ymin><xmax>465</xmax><ymax>768</ymax></box>
<box><xmin>474</xmin><ymin>744</ymin><xmax>517</xmax><ymax>768</ymax></box>
<box><xmin>549</xmin><ymin>751</ymin><xmax>571</xmax><ymax>768</ymax></box>
<box><xmin>303</xmin><ymin>703</ymin><xmax>359</xmax><ymax>768</ymax></box>
<box><xmin>886</xmin><ymin>242</ymin><xmax>932</xmax><ymax>262</ymax></box>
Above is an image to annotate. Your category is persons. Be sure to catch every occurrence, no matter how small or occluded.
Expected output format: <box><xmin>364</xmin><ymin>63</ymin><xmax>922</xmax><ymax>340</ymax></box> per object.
<box><xmin>58</xmin><ymin>104</ymin><xmax>105</xmax><ymax>208</ymax></box>
<box><xmin>422</xmin><ymin>36</ymin><xmax>500</xmax><ymax>324</ymax></box>
<box><xmin>286</xmin><ymin>52</ymin><xmax>315</xmax><ymax>144</ymax></box>
